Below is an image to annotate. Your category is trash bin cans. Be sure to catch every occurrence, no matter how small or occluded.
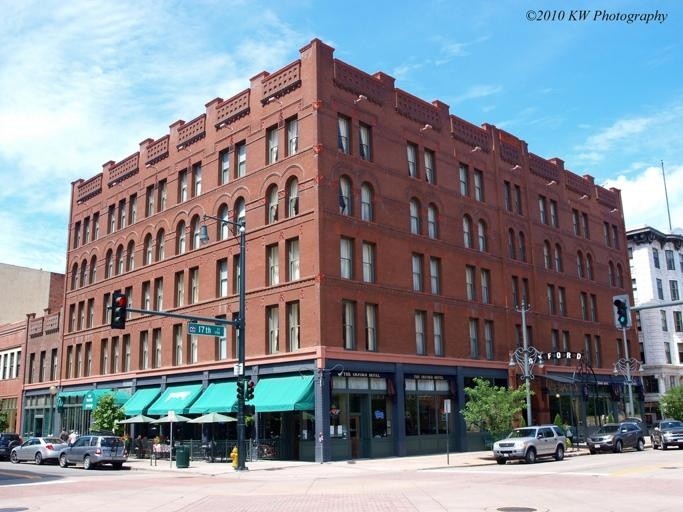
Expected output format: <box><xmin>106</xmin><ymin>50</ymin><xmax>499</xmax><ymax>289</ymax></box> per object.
<box><xmin>174</xmin><ymin>445</ymin><xmax>189</xmax><ymax>468</ymax></box>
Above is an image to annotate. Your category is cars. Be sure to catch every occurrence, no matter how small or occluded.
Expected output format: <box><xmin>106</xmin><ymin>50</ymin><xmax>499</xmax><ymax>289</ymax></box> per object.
<box><xmin>10</xmin><ymin>437</ymin><xmax>68</xmax><ymax>464</ymax></box>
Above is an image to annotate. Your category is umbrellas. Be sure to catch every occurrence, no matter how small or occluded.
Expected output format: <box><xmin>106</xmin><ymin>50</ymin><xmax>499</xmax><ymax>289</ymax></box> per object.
<box><xmin>149</xmin><ymin>414</ymin><xmax>192</xmax><ymax>468</ymax></box>
<box><xmin>115</xmin><ymin>414</ymin><xmax>159</xmax><ymax>437</ymax></box>
<box><xmin>186</xmin><ymin>413</ymin><xmax>238</xmax><ymax>462</ymax></box>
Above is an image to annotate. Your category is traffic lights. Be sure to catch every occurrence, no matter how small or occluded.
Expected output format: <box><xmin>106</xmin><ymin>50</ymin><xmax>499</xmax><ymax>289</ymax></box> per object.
<box><xmin>246</xmin><ymin>380</ymin><xmax>254</xmax><ymax>399</ymax></box>
<box><xmin>613</xmin><ymin>299</ymin><xmax>632</xmax><ymax>329</ymax></box>
<box><xmin>236</xmin><ymin>381</ymin><xmax>244</xmax><ymax>399</ymax></box>
<box><xmin>110</xmin><ymin>293</ymin><xmax>128</xmax><ymax>329</ymax></box>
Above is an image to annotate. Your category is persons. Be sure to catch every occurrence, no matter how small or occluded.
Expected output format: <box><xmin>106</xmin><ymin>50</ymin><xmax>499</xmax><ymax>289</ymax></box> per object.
<box><xmin>47</xmin><ymin>432</ymin><xmax>54</xmax><ymax>437</ymax></box>
<box><xmin>563</xmin><ymin>421</ymin><xmax>575</xmax><ymax>451</ymax></box>
<box><xmin>135</xmin><ymin>435</ymin><xmax>143</xmax><ymax>458</ymax></box>
<box><xmin>124</xmin><ymin>435</ymin><xmax>129</xmax><ymax>456</ymax></box>
<box><xmin>141</xmin><ymin>434</ymin><xmax>148</xmax><ymax>458</ymax></box>
<box><xmin>18</xmin><ymin>433</ymin><xmax>23</xmax><ymax>444</ymax></box>
<box><xmin>59</xmin><ymin>428</ymin><xmax>79</xmax><ymax>445</ymax></box>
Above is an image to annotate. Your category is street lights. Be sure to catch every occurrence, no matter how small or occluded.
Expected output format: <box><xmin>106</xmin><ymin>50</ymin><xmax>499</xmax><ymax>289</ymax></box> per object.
<box><xmin>612</xmin><ymin>359</ymin><xmax>643</xmax><ymax>418</ymax></box>
<box><xmin>199</xmin><ymin>214</ymin><xmax>246</xmax><ymax>472</ymax></box>
<box><xmin>508</xmin><ymin>348</ymin><xmax>544</xmax><ymax>427</ymax></box>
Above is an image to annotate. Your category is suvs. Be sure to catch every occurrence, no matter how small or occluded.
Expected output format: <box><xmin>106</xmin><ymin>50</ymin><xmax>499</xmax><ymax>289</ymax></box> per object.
<box><xmin>648</xmin><ymin>420</ymin><xmax>683</xmax><ymax>450</ymax></box>
<box><xmin>492</xmin><ymin>425</ymin><xmax>566</xmax><ymax>464</ymax></box>
<box><xmin>58</xmin><ymin>436</ymin><xmax>127</xmax><ymax>470</ymax></box>
<box><xmin>0</xmin><ymin>433</ymin><xmax>20</xmax><ymax>456</ymax></box>
<box><xmin>586</xmin><ymin>422</ymin><xmax>645</xmax><ymax>452</ymax></box>
